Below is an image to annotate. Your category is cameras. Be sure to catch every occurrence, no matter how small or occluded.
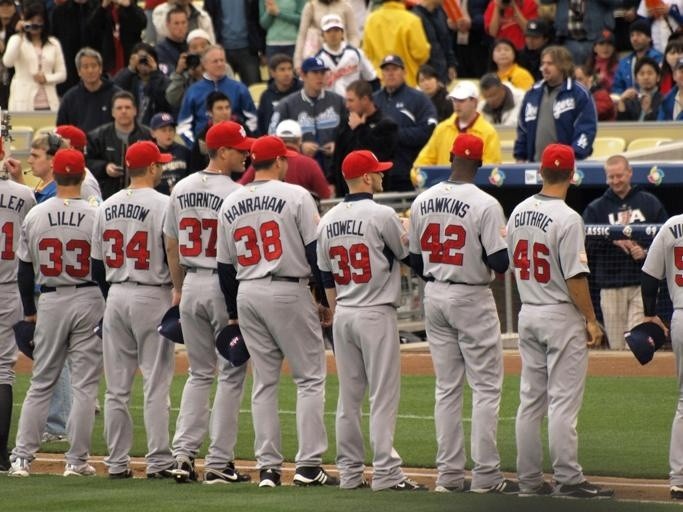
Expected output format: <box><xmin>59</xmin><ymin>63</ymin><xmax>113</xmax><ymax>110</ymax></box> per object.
<box><xmin>634</xmin><ymin>93</ymin><xmax>647</xmax><ymax>101</ymax></box>
<box><xmin>23</xmin><ymin>23</ymin><xmax>44</xmax><ymax>32</ymax></box>
<box><xmin>186</xmin><ymin>54</ymin><xmax>200</xmax><ymax>69</ymax></box>
<box><xmin>139</xmin><ymin>54</ymin><xmax>148</xmax><ymax>64</ymax></box>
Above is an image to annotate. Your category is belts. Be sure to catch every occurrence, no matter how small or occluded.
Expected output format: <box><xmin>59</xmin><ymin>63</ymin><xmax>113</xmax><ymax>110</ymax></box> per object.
<box><xmin>269</xmin><ymin>274</ymin><xmax>300</xmax><ymax>285</ymax></box>
<box><xmin>187</xmin><ymin>266</ymin><xmax>219</xmax><ymax>275</ymax></box>
<box><xmin>38</xmin><ymin>281</ymin><xmax>100</xmax><ymax>294</ymax></box>
<box><xmin>109</xmin><ymin>276</ymin><xmax>173</xmax><ymax>288</ymax></box>
<box><xmin>426</xmin><ymin>276</ymin><xmax>489</xmax><ymax>286</ymax></box>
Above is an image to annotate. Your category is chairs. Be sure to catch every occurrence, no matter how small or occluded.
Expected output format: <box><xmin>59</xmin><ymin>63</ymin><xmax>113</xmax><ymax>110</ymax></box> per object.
<box><xmin>231</xmin><ymin>61</ymin><xmax>269</xmax><ymax>108</ymax></box>
<box><xmin>583</xmin><ymin>137</ymin><xmax>671</xmax><ymax>162</ymax></box>
<box><xmin>0</xmin><ymin>124</ymin><xmax>59</xmax><ymax>158</ymax></box>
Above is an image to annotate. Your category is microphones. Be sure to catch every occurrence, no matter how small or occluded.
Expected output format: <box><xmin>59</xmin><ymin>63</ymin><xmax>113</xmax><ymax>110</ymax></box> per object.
<box><xmin>24</xmin><ymin>170</ymin><xmax>32</xmax><ymax>174</ymax></box>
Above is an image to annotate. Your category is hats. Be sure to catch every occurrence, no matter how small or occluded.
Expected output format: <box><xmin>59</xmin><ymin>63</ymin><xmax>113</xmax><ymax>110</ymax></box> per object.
<box><xmin>300</xmin><ymin>56</ymin><xmax>332</xmax><ymax>73</ymax></box>
<box><xmin>542</xmin><ymin>143</ymin><xmax>574</xmax><ymax>172</ymax></box>
<box><xmin>379</xmin><ymin>54</ymin><xmax>404</xmax><ymax>68</ymax></box>
<box><xmin>341</xmin><ymin>151</ymin><xmax>392</xmax><ymax>180</ymax></box>
<box><xmin>12</xmin><ymin>319</ymin><xmax>36</xmax><ymax>360</ymax></box>
<box><xmin>186</xmin><ymin>28</ymin><xmax>212</xmax><ymax>46</ymax></box>
<box><xmin>157</xmin><ymin>304</ymin><xmax>186</xmax><ymax>345</ymax></box>
<box><xmin>215</xmin><ymin>323</ymin><xmax>250</xmax><ymax>365</ymax></box>
<box><xmin>52</xmin><ymin>151</ymin><xmax>84</xmax><ymax>177</ymax></box>
<box><xmin>451</xmin><ymin>134</ymin><xmax>484</xmax><ymax>162</ymax></box>
<box><xmin>205</xmin><ymin>118</ymin><xmax>304</xmax><ymax>163</ymax></box>
<box><xmin>596</xmin><ymin>29</ymin><xmax>616</xmax><ymax>45</ymax></box>
<box><xmin>525</xmin><ymin>19</ymin><xmax>548</xmax><ymax>36</ymax></box>
<box><xmin>125</xmin><ymin>140</ymin><xmax>173</xmax><ymax>170</ymax></box>
<box><xmin>625</xmin><ymin>321</ymin><xmax>668</xmax><ymax>366</ymax></box>
<box><xmin>444</xmin><ymin>79</ymin><xmax>481</xmax><ymax>102</ymax></box>
<box><xmin>57</xmin><ymin>126</ymin><xmax>86</xmax><ymax>150</ymax></box>
<box><xmin>149</xmin><ymin>112</ymin><xmax>177</xmax><ymax>128</ymax></box>
<box><xmin>320</xmin><ymin>14</ymin><xmax>344</xmax><ymax>31</ymax></box>
<box><xmin>675</xmin><ymin>57</ymin><xmax>683</xmax><ymax>69</ymax></box>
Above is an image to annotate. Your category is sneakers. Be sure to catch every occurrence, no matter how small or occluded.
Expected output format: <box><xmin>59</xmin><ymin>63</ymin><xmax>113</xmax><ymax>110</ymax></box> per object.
<box><xmin>8</xmin><ymin>432</ymin><xmax>251</xmax><ymax>484</ymax></box>
<box><xmin>670</xmin><ymin>485</ymin><xmax>683</xmax><ymax>505</ymax></box>
<box><xmin>260</xmin><ymin>464</ymin><xmax>615</xmax><ymax>500</ymax></box>
